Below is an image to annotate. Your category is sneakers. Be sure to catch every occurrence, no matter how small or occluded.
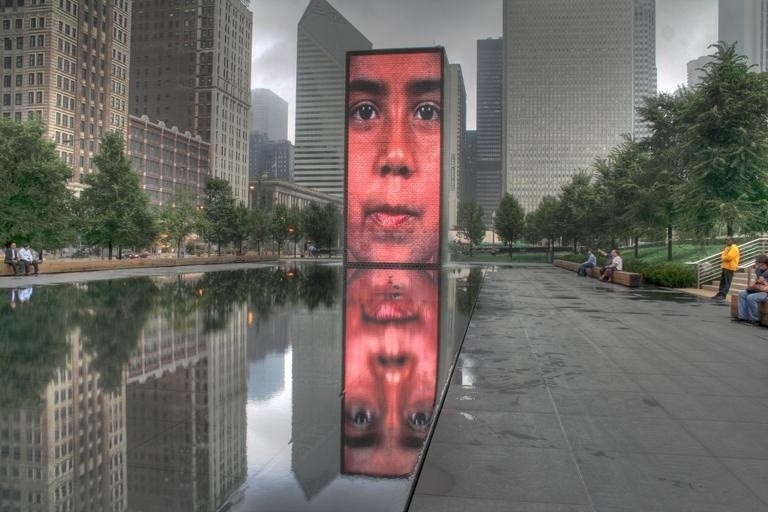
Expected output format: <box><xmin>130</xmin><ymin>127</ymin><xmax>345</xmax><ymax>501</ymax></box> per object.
<box><xmin>713</xmin><ymin>293</ymin><xmax>726</xmax><ymax>299</ymax></box>
<box><xmin>732</xmin><ymin>317</ymin><xmax>759</xmax><ymax>326</ymax></box>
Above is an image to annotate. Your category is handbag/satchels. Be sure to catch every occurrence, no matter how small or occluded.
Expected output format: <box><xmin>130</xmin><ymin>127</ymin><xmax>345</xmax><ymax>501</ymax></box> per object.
<box><xmin>746</xmin><ymin>283</ymin><xmax>768</xmax><ymax>292</ymax></box>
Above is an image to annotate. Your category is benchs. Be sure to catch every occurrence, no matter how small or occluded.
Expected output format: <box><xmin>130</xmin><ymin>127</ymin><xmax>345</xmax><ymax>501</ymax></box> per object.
<box><xmin>731</xmin><ymin>293</ymin><xmax>768</xmax><ymax>326</ymax></box>
<box><xmin>0</xmin><ymin>254</ymin><xmax>280</xmax><ymax>277</ymax></box>
<box><xmin>553</xmin><ymin>259</ymin><xmax>641</xmax><ymax>287</ymax></box>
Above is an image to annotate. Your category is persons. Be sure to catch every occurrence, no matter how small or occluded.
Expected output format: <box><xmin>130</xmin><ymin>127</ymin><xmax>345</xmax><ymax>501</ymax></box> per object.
<box><xmin>7</xmin><ymin>288</ymin><xmax>17</xmax><ymax>309</ymax></box>
<box><xmin>711</xmin><ymin>235</ymin><xmax>739</xmax><ymax>300</ymax></box>
<box><xmin>16</xmin><ymin>287</ymin><xmax>33</xmax><ymax>303</ymax></box>
<box><xmin>736</xmin><ymin>254</ymin><xmax>767</xmax><ymax>325</ymax></box>
<box><xmin>18</xmin><ymin>244</ymin><xmax>39</xmax><ymax>274</ymax></box>
<box><xmin>598</xmin><ymin>247</ymin><xmax>615</xmax><ymax>280</ymax></box>
<box><xmin>600</xmin><ymin>250</ymin><xmax>624</xmax><ymax>283</ymax></box>
<box><xmin>576</xmin><ymin>249</ymin><xmax>597</xmax><ymax>275</ymax></box>
<box><xmin>4</xmin><ymin>243</ymin><xmax>26</xmax><ymax>276</ymax></box>
<box><xmin>340</xmin><ymin>272</ymin><xmax>438</xmax><ymax>473</ymax></box>
<box><xmin>349</xmin><ymin>54</ymin><xmax>441</xmax><ymax>262</ymax></box>
